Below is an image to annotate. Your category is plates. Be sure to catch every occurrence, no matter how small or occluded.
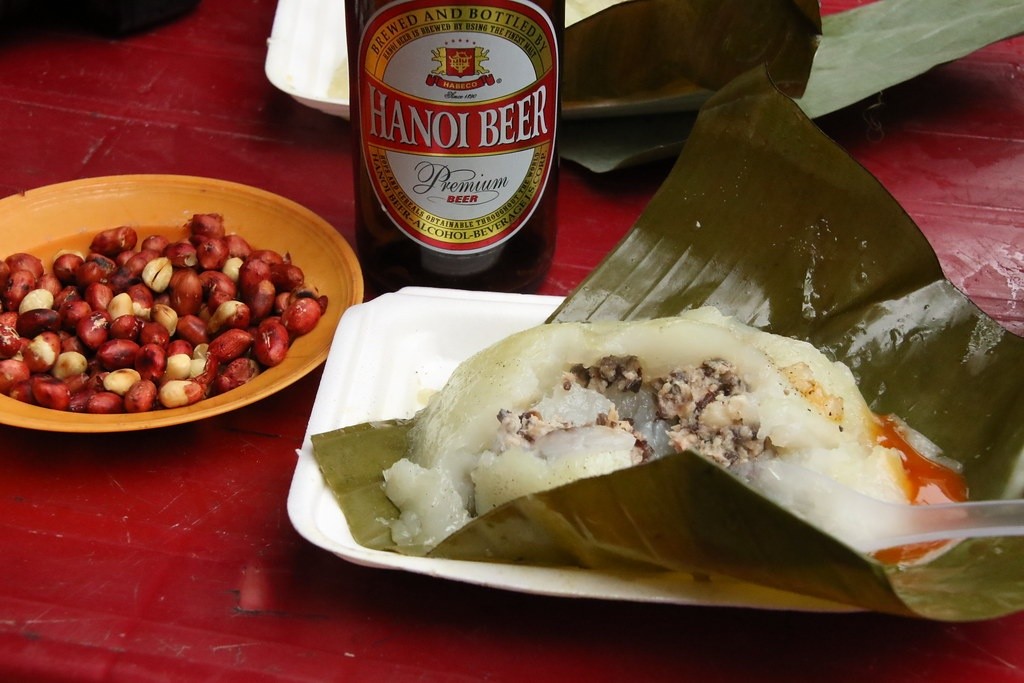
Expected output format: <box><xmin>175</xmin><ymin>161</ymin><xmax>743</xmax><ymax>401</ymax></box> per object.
<box><xmin>287</xmin><ymin>286</ymin><xmax>873</xmax><ymax>613</ymax></box>
<box><xmin>0</xmin><ymin>174</ymin><xmax>364</xmax><ymax>433</ymax></box>
<box><xmin>265</xmin><ymin>0</ymin><xmax>350</xmax><ymax>121</ymax></box>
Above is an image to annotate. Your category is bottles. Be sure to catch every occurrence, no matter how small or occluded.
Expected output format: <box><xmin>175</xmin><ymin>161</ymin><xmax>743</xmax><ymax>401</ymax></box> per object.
<box><xmin>344</xmin><ymin>0</ymin><xmax>566</xmax><ymax>295</ymax></box>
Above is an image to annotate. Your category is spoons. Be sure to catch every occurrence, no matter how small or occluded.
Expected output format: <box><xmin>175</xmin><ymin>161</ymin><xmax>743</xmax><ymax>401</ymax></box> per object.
<box><xmin>729</xmin><ymin>459</ymin><xmax>1024</xmax><ymax>553</ymax></box>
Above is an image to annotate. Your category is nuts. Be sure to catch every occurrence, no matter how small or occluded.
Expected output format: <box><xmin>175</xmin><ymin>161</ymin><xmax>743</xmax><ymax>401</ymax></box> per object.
<box><xmin>0</xmin><ymin>213</ymin><xmax>328</xmax><ymax>415</ymax></box>
